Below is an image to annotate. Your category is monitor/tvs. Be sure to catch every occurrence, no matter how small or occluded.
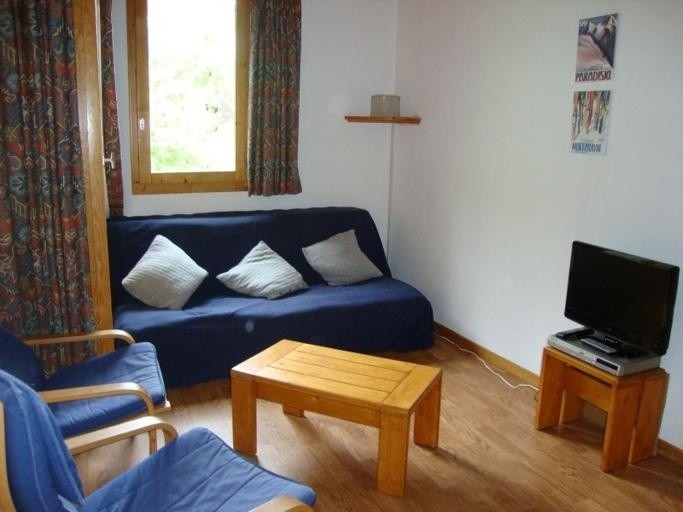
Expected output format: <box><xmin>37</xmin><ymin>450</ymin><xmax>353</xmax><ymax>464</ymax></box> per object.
<box><xmin>563</xmin><ymin>240</ymin><xmax>679</xmax><ymax>356</ymax></box>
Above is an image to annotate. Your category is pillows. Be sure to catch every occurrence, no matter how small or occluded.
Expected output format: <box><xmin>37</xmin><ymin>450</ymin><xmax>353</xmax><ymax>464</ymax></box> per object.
<box><xmin>119</xmin><ymin>227</ymin><xmax>384</xmax><ymax>311</ymax></box>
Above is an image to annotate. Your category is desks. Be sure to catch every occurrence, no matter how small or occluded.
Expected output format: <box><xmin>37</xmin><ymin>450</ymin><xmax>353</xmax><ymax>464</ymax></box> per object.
<box><xmin>532</xmin><ymin>347</ymin><xmax>670</xmax><ymax>474</ymax></box>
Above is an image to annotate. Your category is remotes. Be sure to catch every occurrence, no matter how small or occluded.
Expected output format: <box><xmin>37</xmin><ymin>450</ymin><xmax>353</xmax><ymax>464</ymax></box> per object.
<box><xmin>556</xmin><ymin>326</ymin><xmax>594</xmax><ymax>338</ymax></box>
<box><xmin>580</xmin><ymin>336</ymin><xmax>616</xmax><ymax>354</ymax></box>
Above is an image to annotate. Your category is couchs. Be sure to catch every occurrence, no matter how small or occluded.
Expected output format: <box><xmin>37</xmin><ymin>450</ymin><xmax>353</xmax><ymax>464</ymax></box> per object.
<box><xmin>105</xmin><ymin>205</ymin><xmax>436</xmax><ymax>386</ymax></box>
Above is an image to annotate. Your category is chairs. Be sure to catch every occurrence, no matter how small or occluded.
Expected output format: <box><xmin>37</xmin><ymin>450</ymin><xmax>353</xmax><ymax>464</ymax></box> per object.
<box><xmin>1</xmin><ymin>328</ymin><xmax>171</xmax><ymax>456</ymax></box>
<box><xmin>0</xmin><ymin>369</ymin><xmax>316</xmax><ymax>512</ymax></box>
